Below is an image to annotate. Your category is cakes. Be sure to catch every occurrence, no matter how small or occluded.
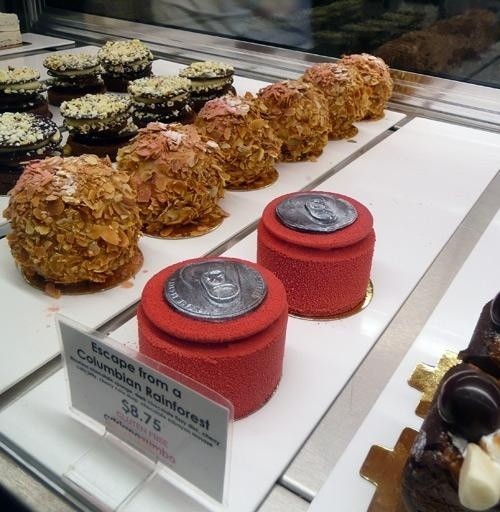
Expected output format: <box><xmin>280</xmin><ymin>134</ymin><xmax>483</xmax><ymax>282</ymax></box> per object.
<box><xmin>399</xmin><ymin>290</ymin><xmax>500</xmax><ymax>512</ymax></box>
<box><xmin>137</xmin><ymin>256</ymin><xmax>289</xmax><ymax>422</ymax></box>
<box><xmin>256</xmin><ymin>190</ymin><xmax>376</xmax><ymax>318</ymax></box>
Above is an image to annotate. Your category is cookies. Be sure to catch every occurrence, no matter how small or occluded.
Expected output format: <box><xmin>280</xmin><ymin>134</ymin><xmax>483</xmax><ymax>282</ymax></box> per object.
<box><xmin>0</xmin><ymin>39</ymin><xmax>236</xmax><ymax>196</ymax></box>
